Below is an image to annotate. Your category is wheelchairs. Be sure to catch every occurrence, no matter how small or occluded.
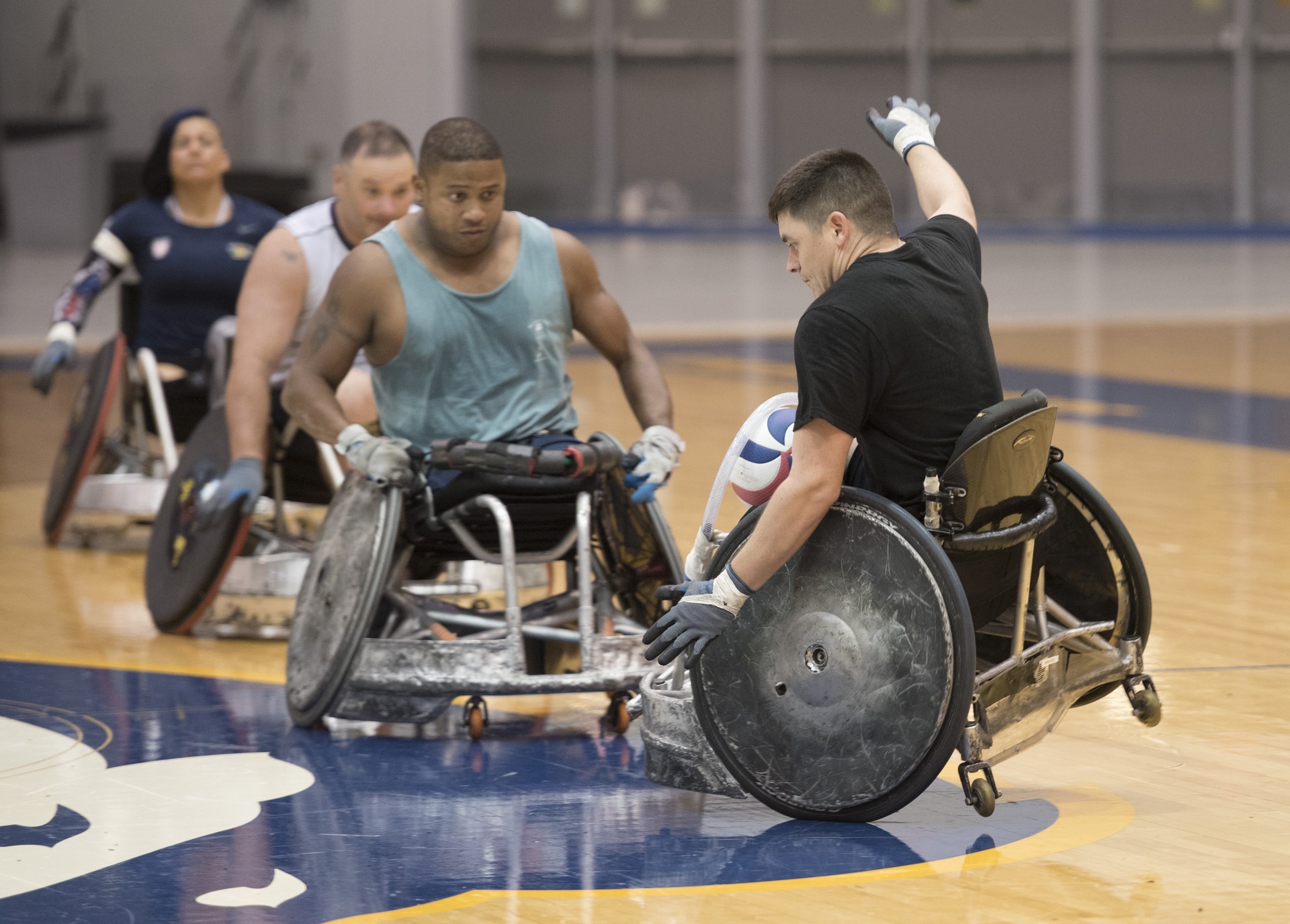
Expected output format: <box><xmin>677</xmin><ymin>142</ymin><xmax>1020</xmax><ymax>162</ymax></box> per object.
<box><xmin>275</xmin><ymin>432</ymin><xmax>691</xmax><ymax>749</ymax></box>
<box><xmin>581</xmin><ymin>378</ymin><xmax>1163</xmax><ymax>831</ymax></box>
<box><xmin>37</xmin><ymin>335</ymin><xmax>217</xmax><ymax>553</ymax></box>
<box><xmin>141</xmin><ymin>383</ymin><xmax>368</xmax><ymax>645</ymax></box>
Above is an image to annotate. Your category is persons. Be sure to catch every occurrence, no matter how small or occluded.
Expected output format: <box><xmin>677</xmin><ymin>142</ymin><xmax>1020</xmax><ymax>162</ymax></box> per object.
<box><xmin>201</xmin><ymin>121</ymin><xmax>423</xmax><ymax>513</ymax></box>
<box><xmin>282</xmin><ymin>118</ymin><xmax>685</xmax><ymax>505</ymax></box>
<box><xmin>643</xmin><ymin>93</ymin><xmax>1006</xmax><ymax>671</ymax></box>
<box><xmin>31</xmin><ymin>108</ymin><xmax>282</xmax><ymax>426</ymax></box>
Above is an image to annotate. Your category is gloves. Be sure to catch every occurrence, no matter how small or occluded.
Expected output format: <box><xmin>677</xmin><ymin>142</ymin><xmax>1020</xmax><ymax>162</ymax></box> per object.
<box><xmin>867</xmin><ymin>95</ymin><xmax>941</xmax><ymax>163</ymax></box>
<box><xmin>643</xmin><ymin>565</ymin><xmax>755</xmax><ymax>670</ymax></box>
<box><xmin>624</xmin><ymin>425</ymin><xmax>686</xmax><ymax>503</ymax></box>
<box><xmin>347</xmin><ymin>436</ymin><xmax>414</xmax><ymax>485</ymax></box>
<box><xmin>31</xmin><ymin>322</ymin><xmax>78</xmax><ymax>395</ymax></box>
<box><xmin>199</xmin><ymin>458</ymin><xmax>265</xmax><ymax>527</ymax></box>
